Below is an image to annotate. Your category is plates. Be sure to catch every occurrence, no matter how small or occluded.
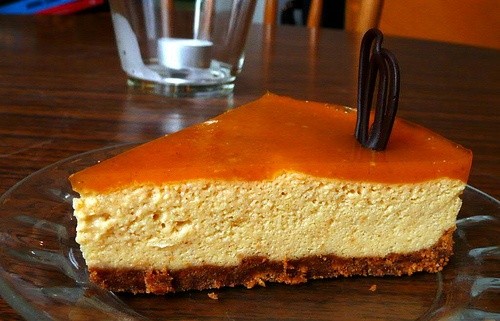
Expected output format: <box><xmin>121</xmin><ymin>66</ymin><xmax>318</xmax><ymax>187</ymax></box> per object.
<box><xmin>0</xmin><ymin>141</ymin><xmax>499</xmax><ymax>321</ymax></box>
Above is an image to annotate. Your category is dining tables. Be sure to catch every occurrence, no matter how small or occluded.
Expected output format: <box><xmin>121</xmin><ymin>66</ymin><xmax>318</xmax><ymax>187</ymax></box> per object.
<box><xmin>1</xmin><ymin>8</ymin><xmax>500</xmax><ymax>320</ymax></box>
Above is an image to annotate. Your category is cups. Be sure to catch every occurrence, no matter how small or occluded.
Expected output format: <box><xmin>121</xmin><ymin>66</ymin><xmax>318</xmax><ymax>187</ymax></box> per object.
<box><xmin>109</xmin><ymin>0</ymin><xmax>256</xmax><ymax>98</ymax></box>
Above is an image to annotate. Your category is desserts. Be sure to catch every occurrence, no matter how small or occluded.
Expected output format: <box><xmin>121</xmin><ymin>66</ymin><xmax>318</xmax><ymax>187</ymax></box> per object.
<box><xmin>67</xmin><ymin>91</ymin><xmax>473</xmax><ymax>296</ymax></box>
